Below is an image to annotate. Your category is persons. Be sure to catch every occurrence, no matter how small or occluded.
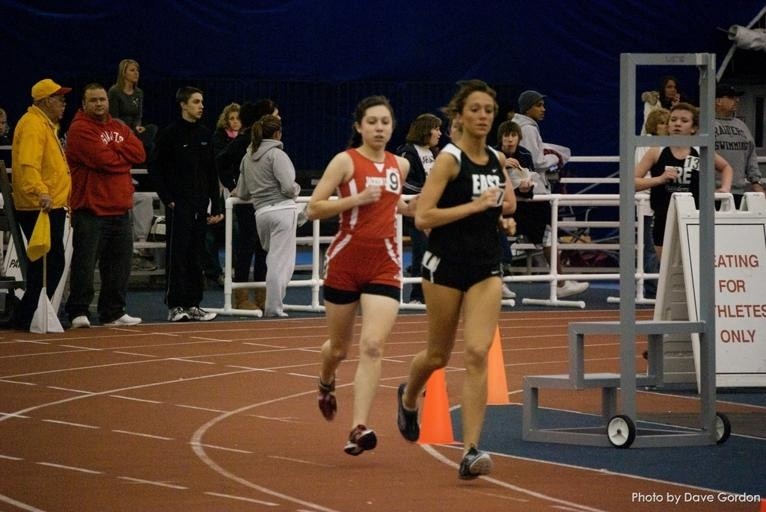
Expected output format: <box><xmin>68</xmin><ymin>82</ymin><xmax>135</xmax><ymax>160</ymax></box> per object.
<box><xmin>398</xmin><ymin>78</ymin><xmax>518</xmax><ymax>478</ymax></box>
<box><xmin>1</xmin><ymin>59</ymin><xmax>301</xmax><ymax>332</ymax></box>
<box><xmin>306</xmin><ymin>95</ymin><xmax>423</xmax><ymax>456</ymax></box>
<box><xmin>635</xmin><ymin>76</ymin><xmax>763</xmax><ymax>303</ymax></box>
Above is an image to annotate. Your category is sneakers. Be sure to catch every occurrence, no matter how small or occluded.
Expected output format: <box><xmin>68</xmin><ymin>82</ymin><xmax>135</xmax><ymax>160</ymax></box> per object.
<box><xmin>343</xmin><ymin>425</ymin><xmax>377</xmax><ymax>456</ymax></box>
<box><xmin>397</xmin><ymin>382</ymin><xmax>420</xmax><ymax>442</ymax></box>
<box><xmin>71</xmin><ymin>316</ymin><xmax>91</xmax><ymax>328</ymax></box>
<box><xmin>318</xmin><ymin>380</ymin><xmax>337</xmax><ymax>423</ymax></box>
<box><xmin>129</xmin><ymin>254</ymin><xmax>157</xmax><ymax>271</ymax></box>
<box><xmin>167</xmin><ymin>306</ymin><xmax>191</xmax><ymax>321</ymax></box>
<box><xmin>557</xmin><ymin>279</ymin><xmax>589</xmax><ymax>300</ymax></box>
<box><xmin>184</xmin><ymin>306</ymin><xmax>218</xmax><ymax>320</ymax></box>
<box><xmin>457</xmin><ymin>442</ymin><xmax>493</xmax><ymax>480</ymax></box>
<box><xmin>500</xmin><ymin>282</ymin><xmax>517</xmax><ymax>299</ymax></box>
<box><xmin>103</xmin><ymin>313</ymin><xmax>142</xmax><ymax>325</ymax></box>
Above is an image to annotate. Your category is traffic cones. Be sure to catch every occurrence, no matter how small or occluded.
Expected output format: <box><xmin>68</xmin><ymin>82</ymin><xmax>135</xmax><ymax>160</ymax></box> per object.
<box><xmin>485</xmin><ymin>325</ymin><xmax>512</xmax><ymax>405</ymax></box>
<box><xmin>414</xmin><ymin>366</ymin><xmax>456</xmax><ymax>448</ymax></box>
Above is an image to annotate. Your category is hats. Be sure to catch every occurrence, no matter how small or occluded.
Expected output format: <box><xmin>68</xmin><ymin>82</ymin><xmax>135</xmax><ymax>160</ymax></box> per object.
<box><xmin>518</xmin><ymin>90</ymin><xmax>547</xmax><ymax>115</ymax></box>
<box><xmin>31</xmin><ymin>79</ymin><xmax>72</xmax><ymax>102</ymax></box>
<box><xmin>716</xmin><ymin>83</ymin><xmax>747</xmax><ymax>97</ymax></box>
